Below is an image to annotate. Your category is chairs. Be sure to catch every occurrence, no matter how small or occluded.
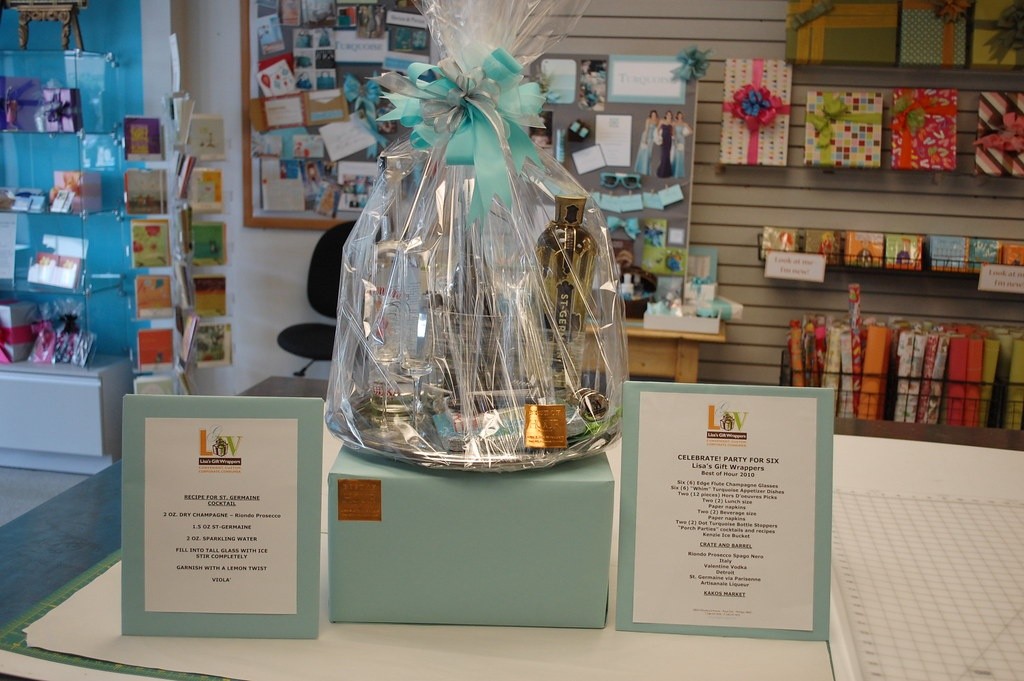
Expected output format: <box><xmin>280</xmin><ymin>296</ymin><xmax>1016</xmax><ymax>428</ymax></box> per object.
<box><xmin>277</xmin><ymin>217</ymin><xmax>380</xmax><ymax>377</ymax></box>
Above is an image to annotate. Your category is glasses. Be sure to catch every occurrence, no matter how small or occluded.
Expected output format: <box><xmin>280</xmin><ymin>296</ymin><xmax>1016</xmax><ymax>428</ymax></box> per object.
<box><xmin>600</xmin><ymin>172</ymin><xmax>640</xmax><ymax>190</ymax></box>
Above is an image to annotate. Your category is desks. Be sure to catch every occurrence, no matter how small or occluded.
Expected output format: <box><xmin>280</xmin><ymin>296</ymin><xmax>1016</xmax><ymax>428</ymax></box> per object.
<box><xmin>1</xmin><ymin>372</ymin><xmax>1024</xmax><ymax>681</ymax></box>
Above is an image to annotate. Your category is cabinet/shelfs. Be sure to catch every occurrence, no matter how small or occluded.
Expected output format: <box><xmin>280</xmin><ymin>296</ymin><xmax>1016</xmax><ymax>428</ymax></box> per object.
<box><xmin>0</xmin><ymin>0</ymin><xmax>143</xmax><ymax>477</ymax></box>
<box><xmin>714</xmin><ymin>1</ymin><xmax>1024</xmax><ymax>428</ymax></box>
